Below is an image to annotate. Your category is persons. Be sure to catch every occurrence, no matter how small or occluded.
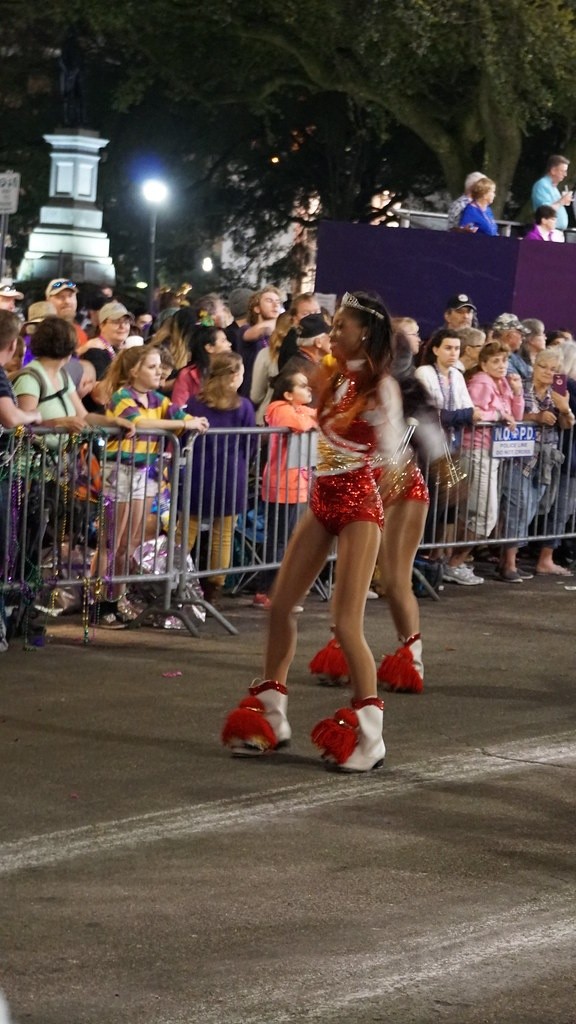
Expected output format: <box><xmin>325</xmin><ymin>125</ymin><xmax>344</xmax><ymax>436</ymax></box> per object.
<box><xmin>442</xmin><ymin>313</ymin><xmax>576</xmax><ymax>585</ymax></box>
<box><xmin>448</xmin><ymin>171</ymin><xmax>500</xmax><ymax>235</ymax></box>
<box><xmin>0</xmin><ymin>276</ymin><xmax>486</xmax><ymax>654</ymax></box>
<box><xmin>221</xmin><ymin>291</ymin><xmax>407</xmax><ymax>773</ymax></box>
<box><xmin>523</xmin><ymin>154</ymin><xmax>575</xmax><ymax>243</ymax></box>
<box><xmin>306</xmin><ymin>376</ymin><xmax>449</xmax><ymax>694</ymax></box>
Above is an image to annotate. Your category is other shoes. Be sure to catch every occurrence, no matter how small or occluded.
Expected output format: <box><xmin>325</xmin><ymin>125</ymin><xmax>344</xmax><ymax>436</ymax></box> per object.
<box><xmin>440</xmin><ymin>560</ymin><xmax>485</xmax><ymax>585</ymax></box>
<box><xmin>252</xmin><ymin>592</ymin><xmax>304</xmax><ymax>613</ymax></box>
<box><xmin>497</xmin><ymin>571</ymin><xmax>524</xmax><ymax>583</ymax></box>
<box><xmin>366</xmin><ymin>589</ymin><xmax>379</xmax><ymax>600</ymax></box>
<box><xmin>535</xmin><ymin>563</ymin><xmax>574</xmax><ymax>576</ymax></box>
<box><xmin>85</xmin><ymin>613</ymin><xmax>127</xmax><ymax>630</ymax></box>
<box><xmin>511</xmin><ymin>569</ymin><xmax>534</xmax><ymax>580</ymax></box>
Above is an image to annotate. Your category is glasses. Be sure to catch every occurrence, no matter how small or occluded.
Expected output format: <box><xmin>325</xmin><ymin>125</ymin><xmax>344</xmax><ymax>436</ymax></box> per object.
<box><xmin>47</xmin><ymin>280</ymin><xmax>77</xmax><ymax>293</ymax></box>
<box><xmin>0</xmin><ymin>285</ymin><xmax>16</xmax><ymax>292</ymax></box>
<box><xmin>404</xmin><ymin>332</ymin><xmax>420</xmax><ymax>336</ymax></box>
<box><xmin>537</xmin><ymin>364</ymin><xmax>558</xmax><ymax>372</ymax></box>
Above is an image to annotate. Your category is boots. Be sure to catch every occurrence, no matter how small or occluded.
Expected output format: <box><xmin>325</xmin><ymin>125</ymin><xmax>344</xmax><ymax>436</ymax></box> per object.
<box><xmin>308</xmin><ymin>624</ymin><xmax>353</xmax><ymax>686</ymax></box>
<box><xmin>225</xmin><ymin>676</ymin><xmax>293</xmax><ymax>757</ymax></box>
<box><xmin>204</xmin><ymin>584</ymin><xmax>222</xmax><ymax>617</ymax></box>
<box><xmin>324</xmin><ymin>695</ymin><xmax>386</xmax><ymax>774</ymax></box>
<box><xmin>376</xmin><ymin>631</ymin><xmax>425</xmax><ymax>694</ymax></box>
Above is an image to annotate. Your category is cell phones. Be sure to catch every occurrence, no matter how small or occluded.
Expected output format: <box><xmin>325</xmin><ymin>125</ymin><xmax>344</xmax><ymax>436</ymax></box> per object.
<box><xmin>553</xmin><ymin>374</ymin><xmax>566</xmax><ymax>397</ymax></box>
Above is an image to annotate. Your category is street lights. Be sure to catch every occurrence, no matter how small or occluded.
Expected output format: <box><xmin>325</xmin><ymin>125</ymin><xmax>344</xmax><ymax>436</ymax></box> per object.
<box><xmin>141</xmin><ymin>177</ymin><xmax>168</xmax><ymax>315</ymax></box>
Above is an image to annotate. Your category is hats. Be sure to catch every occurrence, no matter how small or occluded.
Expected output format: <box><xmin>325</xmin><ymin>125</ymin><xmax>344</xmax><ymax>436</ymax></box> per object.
<box><xmin>226</xmin><ymin>288</ymin><xmax>260</xmax><ymax>319</ymax></box>
<box><xmin>492</xmin><ymin>312</ymin><xmax>532</xmax><ymax>336</ymax></box>
<box><xmin>446</xmin><ymin>293</ymin><xmax>478</xmax><ymax>311</ymax></box>
<box><xmin>45</xmin><ymin>277</ymin><xmax>78</xmax><ymax>299</ymax></box>
<box><xmin>297</xmin><ymin>313</ymin><xmax>334</xmax><ymax>338</ymax></box>
<box><xmin>0</xmin><ymin>278</ymin><xmax>24</xmax><ymax>300</ymax></box>
<box><xmin>97</xmin><ymin>302</ymin><xmax>134</xmax><ymax>324</ymax></box>
<box><xmin>22</xmin><ymin>301</ymin><xmax>58</xmax><ymax>324</ymax></box>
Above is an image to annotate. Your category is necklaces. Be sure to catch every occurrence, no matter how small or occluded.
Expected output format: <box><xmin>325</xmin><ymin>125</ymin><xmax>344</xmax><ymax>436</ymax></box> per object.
<box><xmin>97</xmin><ymin>337</ymin><xmax>117</xmax><ymax>361</ymax></box>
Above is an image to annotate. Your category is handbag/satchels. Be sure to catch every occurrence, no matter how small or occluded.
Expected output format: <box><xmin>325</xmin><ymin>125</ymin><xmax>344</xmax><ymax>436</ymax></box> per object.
<box><xmin>38</xmin><ymin>538</ymin><xmax>114</xmax><ymax>618</ymax></box>
<box><xmin>130</xmin><ymin>535</ymin><xmax>207</xmax><ymax>629</ymax></box>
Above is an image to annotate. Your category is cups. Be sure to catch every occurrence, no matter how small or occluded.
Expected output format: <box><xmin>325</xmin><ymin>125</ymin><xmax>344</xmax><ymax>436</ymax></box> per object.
<box><xmin>561</xmin><ymin>191</ymin><xmax>573</xmax><ymax>206</ymax></box>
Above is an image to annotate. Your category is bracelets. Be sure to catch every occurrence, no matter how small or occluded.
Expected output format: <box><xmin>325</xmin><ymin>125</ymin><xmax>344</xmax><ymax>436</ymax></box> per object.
<box><xmin>558</xmin><ymin>409</ymin><xmax>571</xmax><ymax>417</ymax></box>
<box><xmin>182</xmin><ymin>421</ymin><xmax>187</xmax><ymax>430</ymax></box>
